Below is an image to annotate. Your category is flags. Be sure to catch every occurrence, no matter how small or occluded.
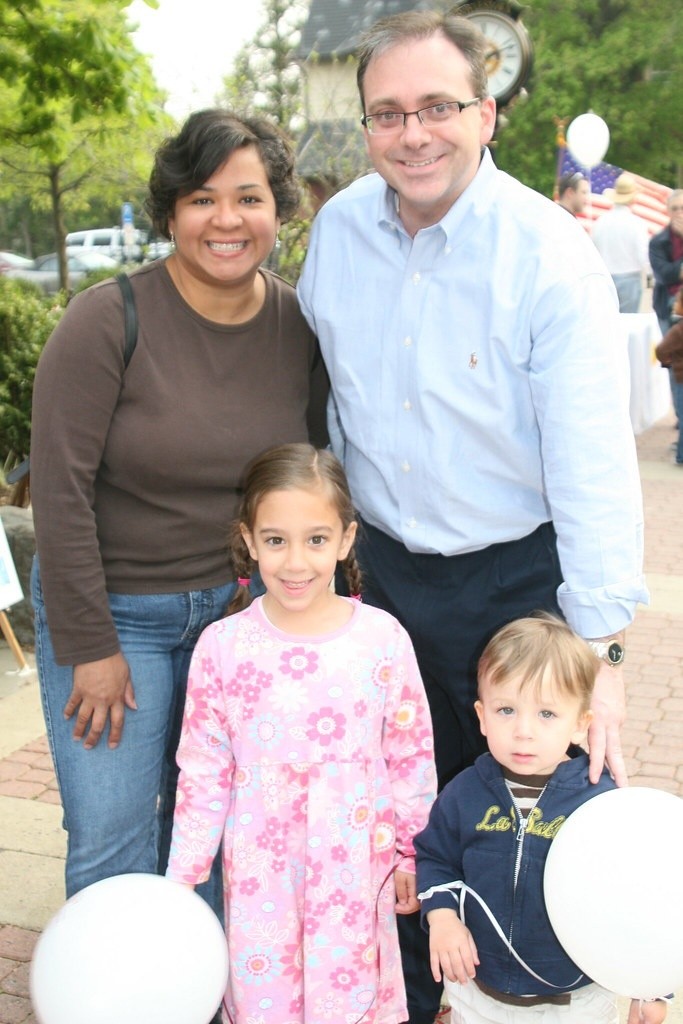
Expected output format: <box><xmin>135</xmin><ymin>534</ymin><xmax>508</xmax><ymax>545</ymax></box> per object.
<box><xmin>556</xmin><ymin>146</ymin><xmax>674</xmax><ymax>244</ymax></box>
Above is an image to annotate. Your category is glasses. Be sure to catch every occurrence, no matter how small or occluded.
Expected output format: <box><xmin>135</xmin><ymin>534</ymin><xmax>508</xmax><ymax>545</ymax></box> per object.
<box><xmin>361</xmin><ymin>98</ymin><xmax>481</xmax><ymax>137</ymax></box>
<box><xmin>670</xmin><ymin>205</ymin><xmax>683</xmax><ymax>212</ymax></box>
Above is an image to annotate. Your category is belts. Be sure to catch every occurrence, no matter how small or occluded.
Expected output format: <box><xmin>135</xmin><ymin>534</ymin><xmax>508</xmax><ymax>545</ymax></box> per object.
<box><xmin>473</xmin><ymin>976</ymin><xmax>570</xmax><ymax>1006</ymax></box>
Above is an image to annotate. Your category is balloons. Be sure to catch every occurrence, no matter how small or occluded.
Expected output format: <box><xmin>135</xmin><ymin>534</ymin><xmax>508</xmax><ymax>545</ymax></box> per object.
<box><xmin>28</xmin><ymin>873</ymin><xmax>227</xmax><ymax>1024</ymax></box>
<box><xmin>541</xmin><ymin>784</ymin><xmax>683</xmax><ymax>1002</ymax></box>
<box><xmin>565</xmin><ymin>111</ymin><xmax>610</xmax><ymax>171</ymax></box>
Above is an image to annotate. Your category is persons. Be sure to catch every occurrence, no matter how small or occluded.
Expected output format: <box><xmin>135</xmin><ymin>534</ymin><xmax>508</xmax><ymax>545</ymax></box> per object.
<box><xmin>27</xmin><ymin>11</ymin><xmax>683</xmax><ymax>1024</ymax></box>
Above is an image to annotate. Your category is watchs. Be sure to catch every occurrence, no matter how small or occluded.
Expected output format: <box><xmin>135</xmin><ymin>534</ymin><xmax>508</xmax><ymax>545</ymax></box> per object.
<box><xmin>588</xmin><ymin>639</ymin><xmax>626</xmax><ymax>668</ymax></box>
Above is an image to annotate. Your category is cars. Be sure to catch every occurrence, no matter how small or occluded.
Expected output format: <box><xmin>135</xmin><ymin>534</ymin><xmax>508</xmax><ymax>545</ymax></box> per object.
<box><xmin>0</xmin><ymin>225</ymin><xmax>177</xmax><ymax>302</ymax></box>
